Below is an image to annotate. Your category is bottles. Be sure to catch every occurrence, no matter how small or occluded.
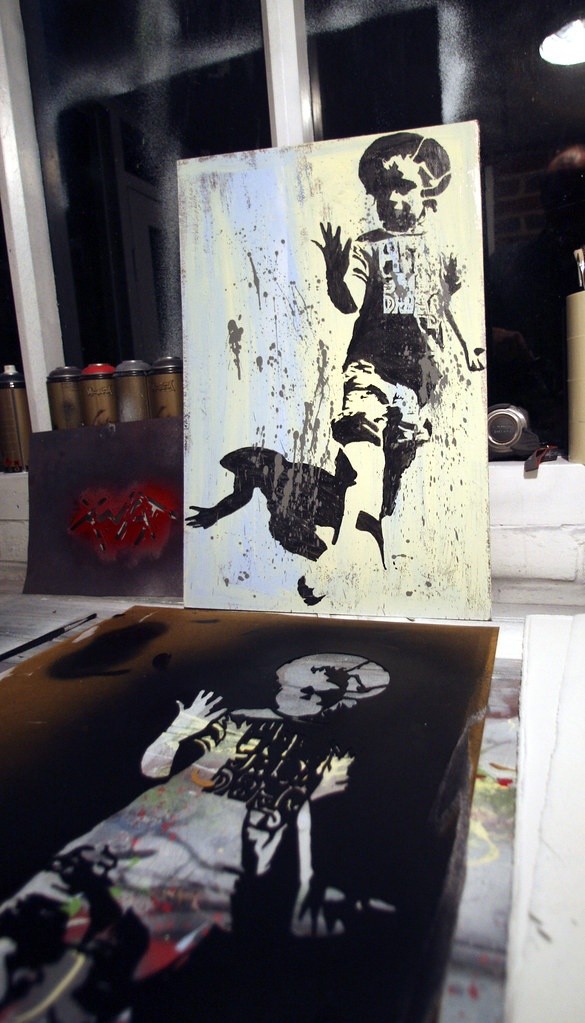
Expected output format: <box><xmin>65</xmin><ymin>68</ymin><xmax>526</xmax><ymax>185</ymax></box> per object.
<box><xmin>0</xmin><ymin>364</ymin><xmax>31</xmax><ymax>471</ymax></box>
<box><xmin>47</xmin><ymin>356</ymin><xmax>184</xmax><ymax>431</ymax></box>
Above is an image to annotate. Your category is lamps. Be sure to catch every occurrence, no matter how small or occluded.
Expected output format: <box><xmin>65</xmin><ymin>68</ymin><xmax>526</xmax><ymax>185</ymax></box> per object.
<box><xmin>539</xmin><ymin>5</ymin><xmax>584</xmax><ymax>69</ymax></box>
